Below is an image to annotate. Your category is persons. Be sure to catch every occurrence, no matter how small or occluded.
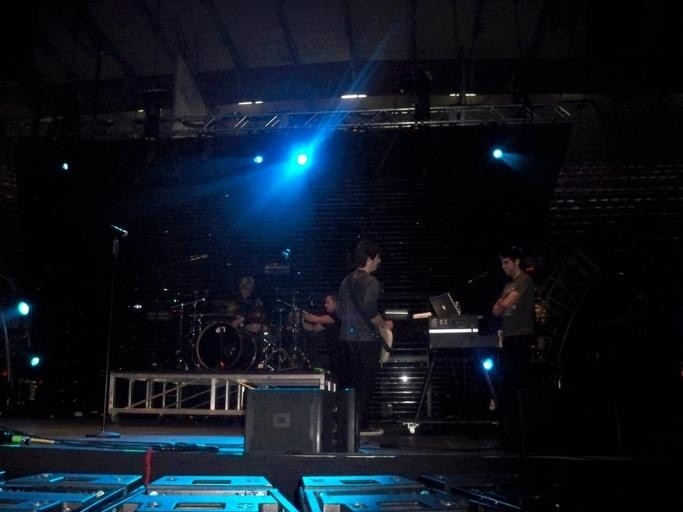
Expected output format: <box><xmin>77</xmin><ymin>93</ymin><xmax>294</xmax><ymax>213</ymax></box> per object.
<box><xmin>221</xmin><ymin>276</ymin><xmax>264</xmax><ymax>426</ymax></box>
<box><xmin>335</xmin><ymin>239</ymin><xmax>395</xmax><ymax>438</ymax></box>
<box><xmin>301</xmin><ymin>293</ymin><xmax>353</xmax><ymax>421</ymax></box>
<box><xmin>492</xmin><ymin>248</ymin><xmax>535</xmax><ymax>446</ymax></box>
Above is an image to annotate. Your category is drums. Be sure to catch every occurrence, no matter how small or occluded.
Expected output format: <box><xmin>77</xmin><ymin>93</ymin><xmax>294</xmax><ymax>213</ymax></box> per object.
<box><xmin>263</xmin><ymin>348</ymin><xmax>311</xmax><ymax>371</ymax></box>
<box><xmin>196</xmin><ymin>322</ymin><xmax>258</xmax><ymax>371</ymax></box>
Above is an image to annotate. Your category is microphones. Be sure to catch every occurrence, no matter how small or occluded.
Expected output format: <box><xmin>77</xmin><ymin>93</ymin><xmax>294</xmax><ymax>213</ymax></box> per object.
<box><xmin>190</xmin><ymin>254</ymin><xmax>208</xmax><ymax>262</ymax></box>
<box><xmin>111</xmin><ymin>224</ymin><xmax>129</xmax><ymax>238</ymax></box>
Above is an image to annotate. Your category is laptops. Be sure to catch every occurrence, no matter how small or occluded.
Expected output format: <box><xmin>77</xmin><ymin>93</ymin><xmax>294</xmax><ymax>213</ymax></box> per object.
<box><xmin>428</xmin><ymin>292</ymin><xmax>484</xmax><ymax>319</ymax></box>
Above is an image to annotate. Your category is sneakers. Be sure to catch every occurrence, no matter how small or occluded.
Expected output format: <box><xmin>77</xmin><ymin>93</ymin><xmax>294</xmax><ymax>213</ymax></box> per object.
<box><xmin>360</xmin><ymin>424</ymin><xmax>384</xmax><ymax>436</ymax></box>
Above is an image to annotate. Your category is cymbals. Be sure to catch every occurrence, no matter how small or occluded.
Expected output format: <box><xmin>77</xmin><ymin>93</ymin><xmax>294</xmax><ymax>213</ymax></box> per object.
<box><xmin>246</xmin><ymin>323</ymin><xmax>275</xmax><ymax>334</ymax></box>
<box><xmin>211</xmin><ymin>299</ymin><xmax>241</xmax><ymax>313</ymax></box>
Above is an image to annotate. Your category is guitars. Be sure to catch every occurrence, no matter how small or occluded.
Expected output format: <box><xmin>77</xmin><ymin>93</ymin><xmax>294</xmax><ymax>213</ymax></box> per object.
<box><xmin>375</xmin><ymin>281</ymin><xmax>394</xmax><ymax>364</ymax></box>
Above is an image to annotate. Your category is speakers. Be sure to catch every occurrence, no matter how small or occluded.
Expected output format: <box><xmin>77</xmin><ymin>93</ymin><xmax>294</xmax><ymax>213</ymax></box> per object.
<box><xmin>244</xmin><ymin>386</ymin><xmax>360</xmax><ymax>457</ymax></box>
